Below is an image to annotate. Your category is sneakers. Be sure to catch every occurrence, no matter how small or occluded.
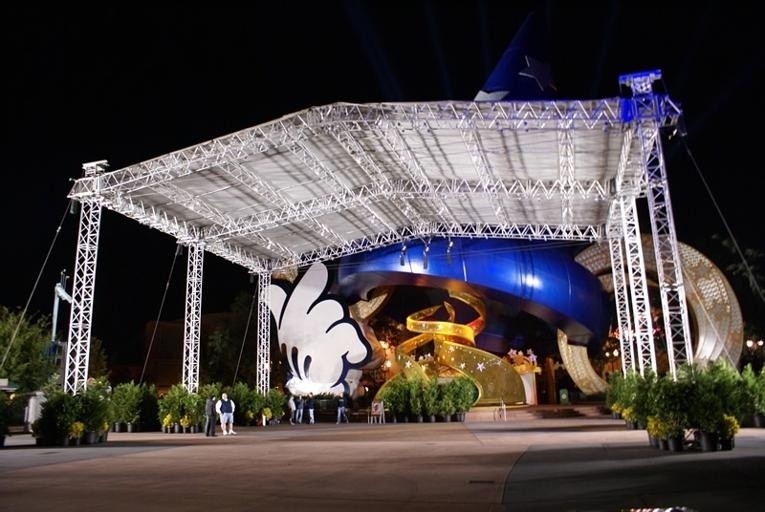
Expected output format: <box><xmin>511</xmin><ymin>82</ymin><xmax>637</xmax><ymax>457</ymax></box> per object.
<box><xmin>222</xmin><ymin>430</ymin><xmax>237</xmax><ymax>436</ymax></box>
<box><xmin>206</xmin><ymin>433</ymin><xmax>218</xmax><ymax>437</ymax></box>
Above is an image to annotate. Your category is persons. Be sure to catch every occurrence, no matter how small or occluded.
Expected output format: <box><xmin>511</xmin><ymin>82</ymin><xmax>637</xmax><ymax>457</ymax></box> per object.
<box><xmin>205</xmin><ymin>393</ymin><xmax>218</xmax><ymax>437</ymax></box>
<box><xmin>336</xmin><ymin>395</ymin><xmax>350</xmax><ymax>425</ymax></box>
<box><xmin>315</xmin><ymin>394</ymin><xmax>321</xmax><ymax>421</ymax></box>
<box><xmin>304</xmin><ymin>391</ymin><xmax>316</xmax><ymax>423</ymax></box>
<box><xmin>294</xmin><ymin>394</ymin><xmax>305</xmax><ymax>424</ymax></box>
<box><xmin>286</xmin><ymin>393</ymin><xmax>296</xmax><ymax>426</ymax></box>
<box><xmin>215</xmin><ymin>391</ymin><xmax>237</xmax><ymax>435</ymax></box>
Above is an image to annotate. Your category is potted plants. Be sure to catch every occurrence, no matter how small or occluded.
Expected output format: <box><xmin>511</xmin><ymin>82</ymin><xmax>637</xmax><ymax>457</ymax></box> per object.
<box><xmin>605</xmin><ymin>359</ymin><xmax>763</xmax><ymax>454</ymax></box>
<box><xmin>381</xmin><ymin>375</ymin><xmax>475</xmax><ymax>422</ymax></box>
<box><xmin>22</xmin><ymin>379</ymin><xmax>287</xmax><ymax>448</ymax></box>
